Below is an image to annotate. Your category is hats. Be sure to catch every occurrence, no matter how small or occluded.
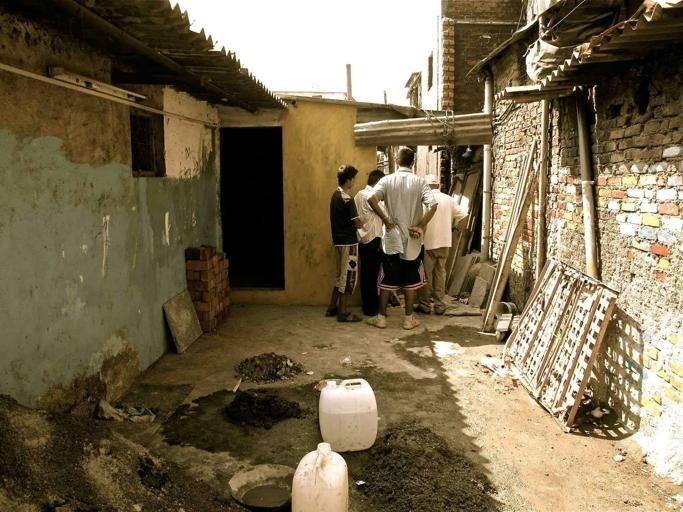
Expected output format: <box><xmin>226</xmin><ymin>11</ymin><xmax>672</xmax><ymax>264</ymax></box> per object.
<box><xmin>425</xmin><ymin>175</ymin><xmax>441</xmax><ymax>185</ymax></box>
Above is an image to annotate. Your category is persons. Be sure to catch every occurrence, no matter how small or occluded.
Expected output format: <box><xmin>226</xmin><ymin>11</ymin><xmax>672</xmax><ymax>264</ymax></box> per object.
<box><xmin>366</xmin><ymin>146</ymin><xmax>438</xmax><ymax>330</ymax></box>
<box><xmin>413</xmin><ymin>174</ymin><xmax>468</xmax><ymax>315</ymax></box>
<box><xmin>326</xmin><ymin>165</ymin><xmax>362</xmax><ymax>322</ymax></box>
<box><xmin>354</xmin><ymin>170</ymin><xmax>389</xmax><ymax>318</ymax></box>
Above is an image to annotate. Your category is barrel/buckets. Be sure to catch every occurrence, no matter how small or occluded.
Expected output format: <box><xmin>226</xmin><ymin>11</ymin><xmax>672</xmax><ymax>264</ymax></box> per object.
<box><xmin>319</xmin><ymin>377</ymin><xmax>378</xmax><ymax>453</ymax></box>
<box><xmin>290</xmin><ymin>441</ymin><xmax>349</xmax><ymax>512</ymax></box>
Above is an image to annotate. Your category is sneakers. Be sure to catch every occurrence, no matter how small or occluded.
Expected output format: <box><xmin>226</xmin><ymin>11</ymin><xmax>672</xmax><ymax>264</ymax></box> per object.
<box><xmin>403</xmin><ymin>318</ymin><xmax>421</xmax><ymax>329</ymax></box>
<box><xmin>338</xmin><ymin>313</ymin><xmax>361</xmax><ymax>321</ymax></box>
<box><xmin>413</xmin><ymin>304</ymin><xmax>431</xmax><ymax>314</ymax></box>
<box><xmin>325</xmin><ymin>307</ymin><xmax>338</xmax><ymax>317</ymax></box>
<box><xmin>435</xmin><ymin>312</ymin><xmax>442</xmax><ymax>315</ymax></box>
<box><xmin>368</xmin><ymin>314</ymin><xmax>386</xmax><ymax>328</ymax></box>
